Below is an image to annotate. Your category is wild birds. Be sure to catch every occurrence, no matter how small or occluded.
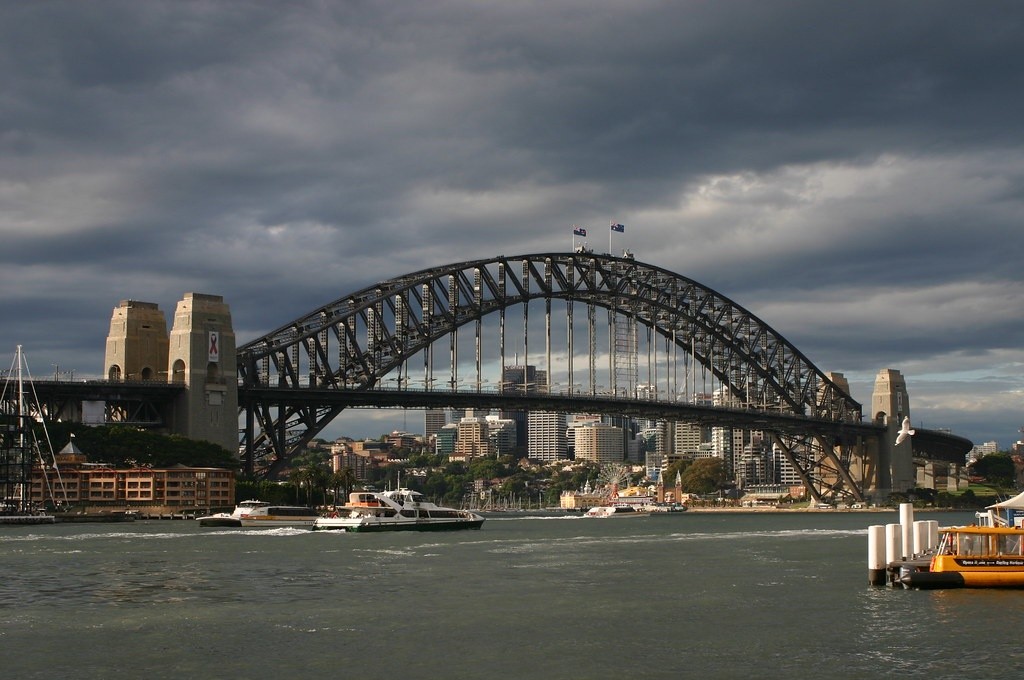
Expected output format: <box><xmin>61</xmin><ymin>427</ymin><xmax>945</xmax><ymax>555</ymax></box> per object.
<box><xmin>895</xmin><ymin>416</ymin><xmax>915</xmax><ymax>446</ymax></box>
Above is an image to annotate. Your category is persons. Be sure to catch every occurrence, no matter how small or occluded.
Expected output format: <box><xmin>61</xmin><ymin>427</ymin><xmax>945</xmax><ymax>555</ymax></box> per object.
<box><xmin>964</xmin><ymin>522</ymin><xmax>1006</xmax><ymax>555</ymax></box>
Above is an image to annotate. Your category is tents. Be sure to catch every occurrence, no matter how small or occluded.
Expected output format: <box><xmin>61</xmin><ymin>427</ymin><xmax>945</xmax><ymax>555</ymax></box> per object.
<box><xmin>985</xmin><ymin>491</ymin><xmax>1024</xmax><ymax>511</ymax></box>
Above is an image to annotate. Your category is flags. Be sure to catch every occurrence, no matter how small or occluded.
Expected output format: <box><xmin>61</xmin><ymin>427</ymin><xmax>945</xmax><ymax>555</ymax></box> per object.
<box><xmin>611</xmin><ymin>221</ymin><xmax>624</xmax><ymax>232</ymax></box>
<box><xmin>573</xmin><ymin>226</ymin><xmax>587</xmax><ymax>237</ymax></box>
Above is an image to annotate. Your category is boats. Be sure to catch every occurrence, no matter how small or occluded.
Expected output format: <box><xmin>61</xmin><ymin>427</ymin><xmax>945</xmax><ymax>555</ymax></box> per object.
<box><xmin>311</xmin><ymin>471</ymin><xmax>486</xmax><ymax>532</ymax></box>
<box><xmin>194</xmin><ymin>497</ymin><xmax>336</xmax><ymax>528</ymax></box>
<box><xmin>887</xmin><ymin>521</ymin><xmax>1024</xmax><ymax>590</ymax></box>
<box><xmin>583</xmin><ymin>503</ymin><xmax>651</xmax><ymax>518</ymax></box>
